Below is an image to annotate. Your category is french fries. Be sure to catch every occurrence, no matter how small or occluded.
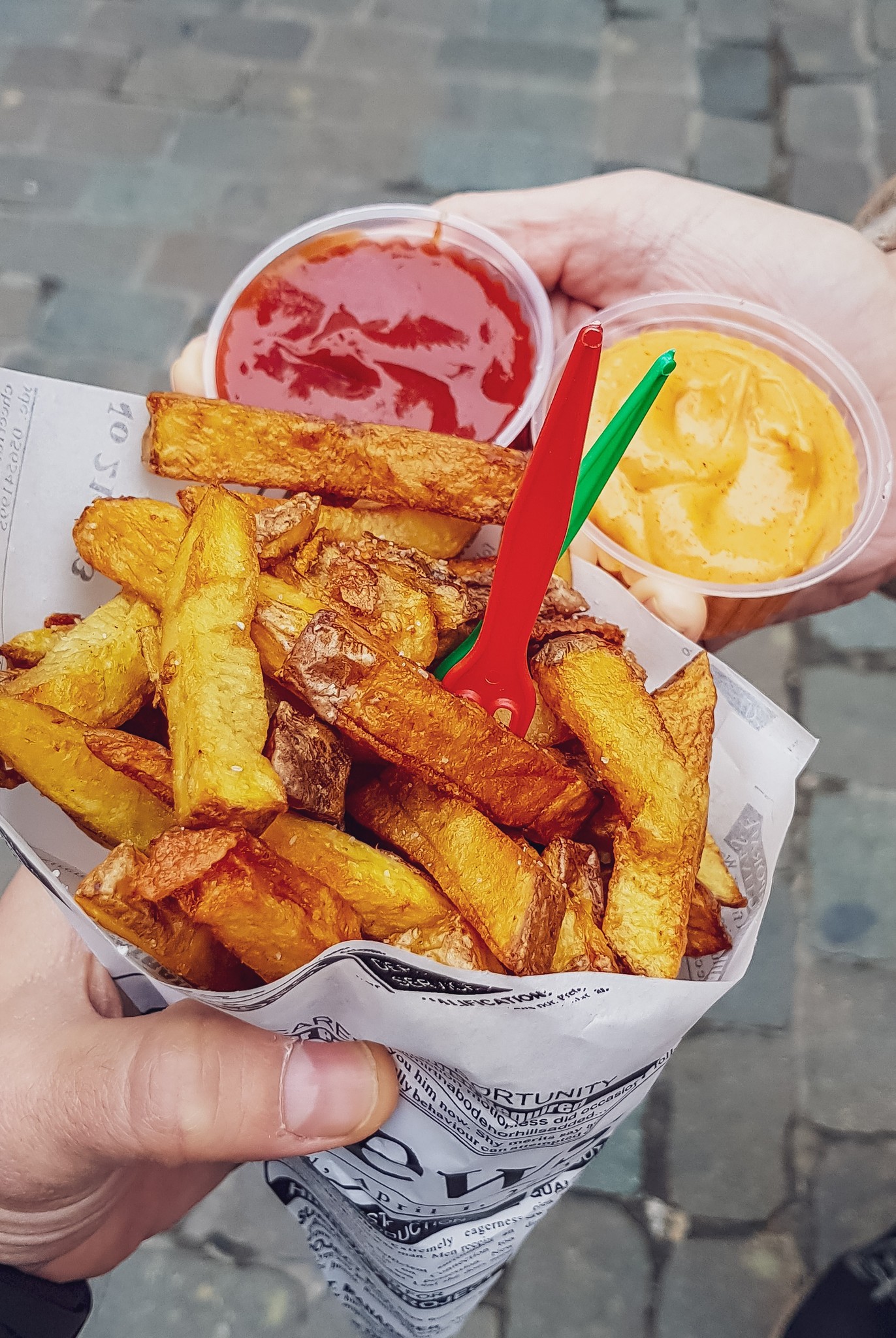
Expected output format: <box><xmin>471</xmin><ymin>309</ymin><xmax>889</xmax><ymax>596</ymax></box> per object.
<box><xmin>1</xmin><ymin>390</ymin><xmax>746</xmax><ymax>990</ymax></box>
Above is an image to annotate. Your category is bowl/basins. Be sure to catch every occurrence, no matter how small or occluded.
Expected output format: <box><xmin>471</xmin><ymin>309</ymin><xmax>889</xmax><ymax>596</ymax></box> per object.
<box><xmin>203</xmin><ymin>202</ymin><xmax>555</xmax><ymax>447</ymax></box>
<box><xmin>529</xmin><ymin>291</ymin><xmax>894</xmax><ymax>640</ymax></box>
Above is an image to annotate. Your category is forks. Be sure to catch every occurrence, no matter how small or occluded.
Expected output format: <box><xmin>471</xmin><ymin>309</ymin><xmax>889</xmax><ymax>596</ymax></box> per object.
<box><xmin>432</xmin><ymin>323</ymin><xmax>678</xmax><ymax>741</ymax></box>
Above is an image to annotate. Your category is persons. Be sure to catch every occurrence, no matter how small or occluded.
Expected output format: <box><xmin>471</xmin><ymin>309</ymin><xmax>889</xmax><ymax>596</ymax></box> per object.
<box><xmin>0</xmin><ymin>866</ymin><xmax>896</xmax><ymax>1338</ymax></box>
<box><xmin>170</xmin><ymin>166</ymin><xmax>896</xmax><ymax>653</ymax></box>
